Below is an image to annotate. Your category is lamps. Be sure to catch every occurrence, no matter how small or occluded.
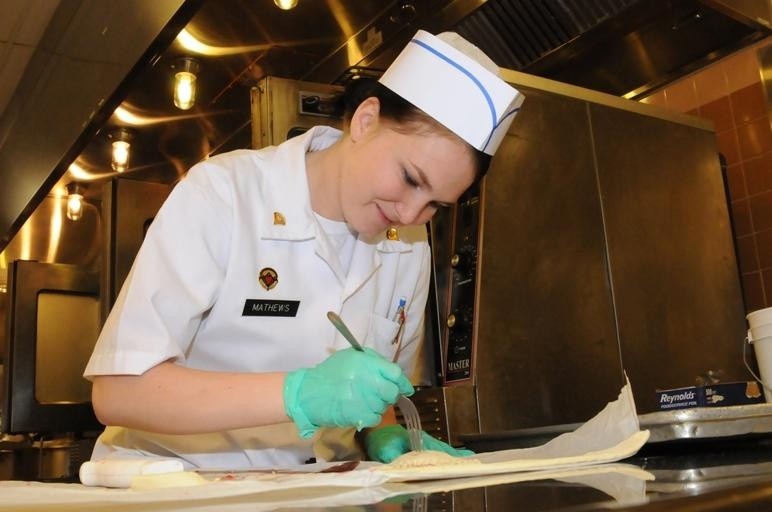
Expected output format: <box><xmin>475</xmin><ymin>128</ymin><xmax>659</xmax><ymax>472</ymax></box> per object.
<box><xmin>111</xmin><ymin>129</ymin><xmax>132</xmax><ymax>174</ymax></box>
<box><xmin>65</xmin><ymin>181</ymin><xmax>86</xmax><ymax>222</ymax></box>
<box><xmin>174</xmin><ymin>57</ymin><xmax>198</xmax><ymax>112</ymax></box>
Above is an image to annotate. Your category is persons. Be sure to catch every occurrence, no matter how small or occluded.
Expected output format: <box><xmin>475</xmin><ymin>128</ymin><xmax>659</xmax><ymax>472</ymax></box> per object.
<box><xmin>81</xmin><ymin>31</ymin><xmax>526</xmax><ymax>473</ymax></box>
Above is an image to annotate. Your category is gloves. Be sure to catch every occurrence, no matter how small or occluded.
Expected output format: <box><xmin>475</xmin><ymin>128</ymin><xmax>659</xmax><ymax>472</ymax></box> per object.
<box><xmin>286</xmin><ymin>344</ymin><xmax>416</xmax><ymax>437</ymax></box>
<box><xmin>362</xmin><ymin>426</ymin><xmax>475</xmax><ymax>465</ymax></box>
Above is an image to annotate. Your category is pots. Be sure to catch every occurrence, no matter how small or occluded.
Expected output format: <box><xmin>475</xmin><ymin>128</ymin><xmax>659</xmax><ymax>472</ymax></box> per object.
<box><xmin>0</xmin><ymin>439</ymin><xmax>87</xmax><ymax>483</ymax></box>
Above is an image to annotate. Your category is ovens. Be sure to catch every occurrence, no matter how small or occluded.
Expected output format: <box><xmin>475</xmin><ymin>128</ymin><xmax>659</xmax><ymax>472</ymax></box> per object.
<box><xmin>393</xmin><ymin>64</ymin><xmax>756</xmax><ymax>449</ymax></box>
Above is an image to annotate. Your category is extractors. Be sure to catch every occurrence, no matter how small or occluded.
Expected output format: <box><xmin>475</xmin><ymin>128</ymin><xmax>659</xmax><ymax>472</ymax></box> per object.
<box><xmin>299</xmin><ymin>0</ymin><xmax>771</xmax><ymax>105</ymax></box>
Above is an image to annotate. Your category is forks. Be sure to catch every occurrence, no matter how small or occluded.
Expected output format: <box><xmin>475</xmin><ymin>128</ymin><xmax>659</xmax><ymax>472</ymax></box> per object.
<box><xmin>326</xmin><ymin>310</ymin><xmax>425</xmax><ymax>452</ymax></box>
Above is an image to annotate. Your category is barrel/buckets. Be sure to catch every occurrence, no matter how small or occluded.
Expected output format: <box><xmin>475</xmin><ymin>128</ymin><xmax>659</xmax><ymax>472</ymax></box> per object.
<box><xmin>744</xmin><ymin>307</ymin><xmax>772</xmax><ymax>403</ymax></box>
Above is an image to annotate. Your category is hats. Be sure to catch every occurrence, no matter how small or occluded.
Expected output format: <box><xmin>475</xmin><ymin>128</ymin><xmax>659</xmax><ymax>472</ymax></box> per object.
<box><xmin>381</xmin><ymin>29</ymin><xmax>527</xmax><ymax>158</ymax></box>
